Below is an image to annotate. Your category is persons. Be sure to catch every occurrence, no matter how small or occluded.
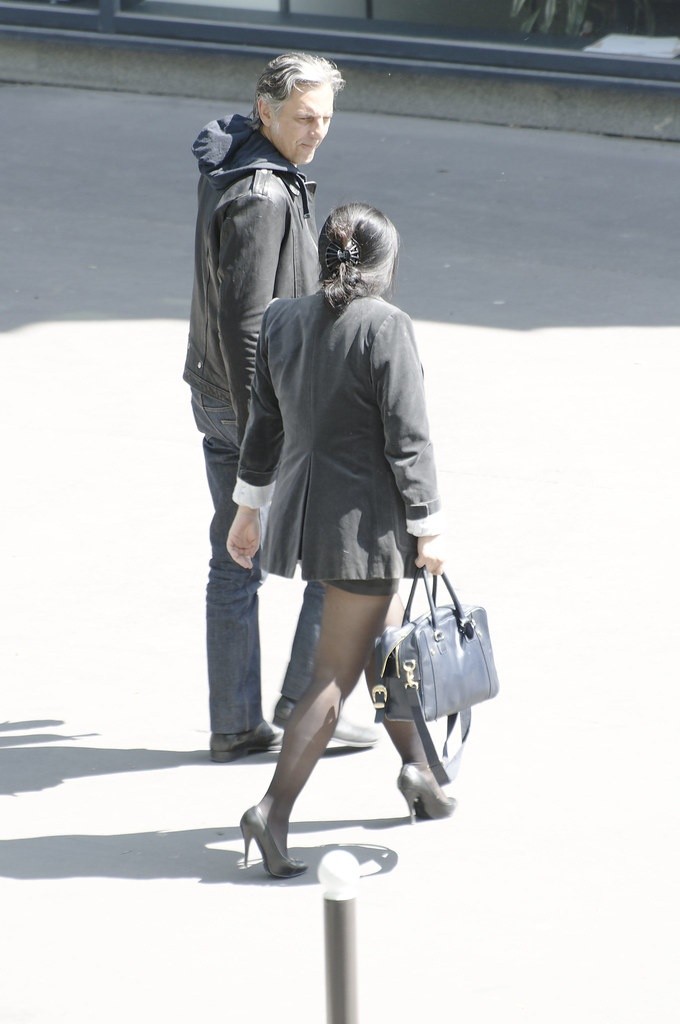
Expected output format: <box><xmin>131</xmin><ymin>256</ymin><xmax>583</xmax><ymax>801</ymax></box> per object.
<box><xmin>182</xmin><ymin>51</ymin><xmax>380</xmax><ymax>761</ymax></box>
<box><xmin>224</xmin><ymin>203</ymin><xmax>459</xmax><ymax>878</ymax></box>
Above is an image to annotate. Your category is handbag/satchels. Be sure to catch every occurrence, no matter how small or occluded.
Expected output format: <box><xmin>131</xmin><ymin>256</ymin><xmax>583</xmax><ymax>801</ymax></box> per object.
<box><xmin>370</xmin><ymin>561</ymin><xmax>499</xmax><ymax>787</ymax></box>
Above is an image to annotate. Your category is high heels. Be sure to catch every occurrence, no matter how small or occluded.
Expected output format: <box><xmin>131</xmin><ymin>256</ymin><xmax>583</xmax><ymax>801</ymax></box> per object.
<box><xmin>241</xmin><ymin>806</ymin><xmax>307</xmax><ymax>878</ymax></box>
<box><xmin>397</xmin><ymin>760</ymin><xmax>457</xmax><ymax>824</ymax></box>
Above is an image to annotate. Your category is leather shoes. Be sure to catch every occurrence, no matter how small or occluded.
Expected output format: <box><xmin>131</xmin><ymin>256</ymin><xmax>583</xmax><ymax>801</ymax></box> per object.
<box><xmin>210</xmin><ymin>722</ymin><xmax>287</xmax><ymax>761</ymax></box>
<box><xmin>273</xmin><ymin>696</ymin><xmax>376</xmax><ymax>746</ymax></box>
